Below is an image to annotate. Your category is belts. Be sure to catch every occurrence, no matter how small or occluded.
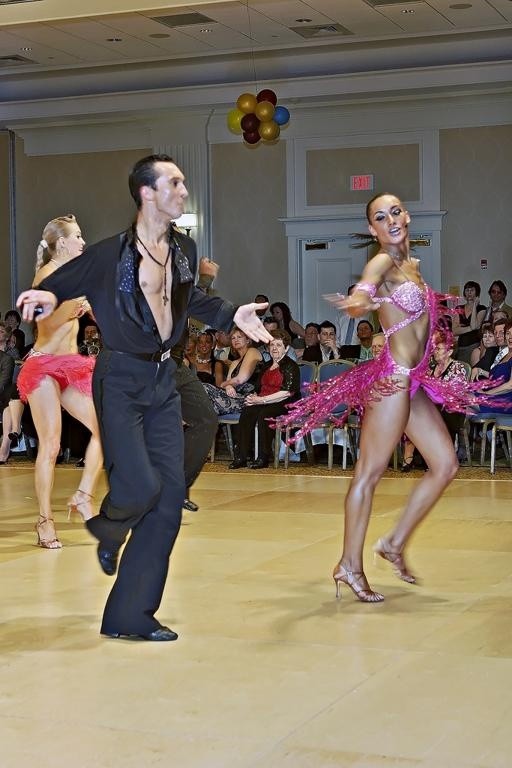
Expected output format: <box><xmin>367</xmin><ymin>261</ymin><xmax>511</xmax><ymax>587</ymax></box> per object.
<box><xmin>105</xmin><ymin>350</ymin><xmax>173</xmax><ymax>363</ymax></box>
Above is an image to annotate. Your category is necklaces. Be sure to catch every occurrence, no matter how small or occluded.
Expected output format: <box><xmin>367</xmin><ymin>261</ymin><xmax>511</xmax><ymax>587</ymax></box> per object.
<box><xmin>136</xmin><ymin>233</ymin><xmax>172</xmax><ymax>307</ymax></box>
<box><xmin>50</xmin><ymin>256</ymin><xmax>64</xmax><ymax>268</ymax></box>
<box><xmin>382</xmin><ymin>246</ymin><xmax>406</xmax><ymax>262</ymax></box>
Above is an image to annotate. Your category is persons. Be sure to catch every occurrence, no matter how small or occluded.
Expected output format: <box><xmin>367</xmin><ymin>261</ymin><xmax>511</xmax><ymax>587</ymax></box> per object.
<box><xmin>14</xmin><ymin>212</ymin><xmax>105</xmax><ymax>550</ymax></box>
<box><xmin>320</xmin><ymin>190</ymin><xmax>463</xmax><ymax>605</ymax></box>
<box><xmin>179</xmin><ymin>254</ymin><xmax>221</xmax><ymax>512</ymax></box>
<box><xmin>2</xmin><ymin>278</ymin><xmax>511</xmax><ymax>474</ymax></box>
<box><xmin>15</xmin><ymin>150</ymin><xmax>276</xmax><ymax>644</ymax></box>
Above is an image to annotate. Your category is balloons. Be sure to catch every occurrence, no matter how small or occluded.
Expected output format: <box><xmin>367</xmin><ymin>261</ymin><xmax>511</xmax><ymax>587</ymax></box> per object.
<box><xmin>226</xmin><ymin>88</ymin><xmax>290</xmax><ymax>145</ymax></box>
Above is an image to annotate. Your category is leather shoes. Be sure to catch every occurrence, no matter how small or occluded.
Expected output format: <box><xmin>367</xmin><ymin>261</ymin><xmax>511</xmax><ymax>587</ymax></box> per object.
<box><xmin>249</xmin><ymin>456</ymin><xmax>269</xmax><ymax>469</ymax></box>
<box><xmin>75</xmin><ymin>457</ymin><xmax>85</xmax><ymax>467</ymax></box>
<box><xmin>229</xmin><ymin>455</ymin><xmax>247</xmax><ymax>468</ymax></box>
<box><xmin>98</xmin><ymin>542</ymin><xmax>120</xmax><ymax>575</ymax></box>
<box><xmin>400</xmin><ymin>457</ymin><xmax>415</xmax><ymax>471</ymax></box>
<box><xmin>182</xmin><ymin>497</ymin><xmax>198</xmax><ymax>512</ymax></box>
<box><xmin>56</xmin><ymin>449</ymin><xmax>64</xmax><ymax>464</ymax></box>
<box><xmin>109</xmin><ymin>625</ymin><xmax>178</xmax><ymax>639</ymax></box>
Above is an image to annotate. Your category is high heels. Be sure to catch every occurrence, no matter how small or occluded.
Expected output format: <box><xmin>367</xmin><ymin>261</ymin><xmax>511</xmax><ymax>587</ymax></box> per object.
<box><xmin>0</xmin><ymin>457</ymin><xmax>8</xmax><ymax>464</ymax></box>
<box><xmin>9</xmin><ymin>429</ymin><xmax>23</xmax><ymax>447</ymax></box>
<box><xmin>66</xmin><ymin>490</ymin><xmax>94</xmax><ymax>522</ymax></box>
<box><xmin>372</xmin><ymin>537</ymin><xmax>417</xmax><ymax>583</ymax></box>
<box><xmin>334</xmin><ymin>563</ymin><xmax>384</xmax><ymax>602</ymax></box>
<box><xmin>35</xmin><ymin>515</ymin><xmax>62</xmax><ymax>549</ymax></box>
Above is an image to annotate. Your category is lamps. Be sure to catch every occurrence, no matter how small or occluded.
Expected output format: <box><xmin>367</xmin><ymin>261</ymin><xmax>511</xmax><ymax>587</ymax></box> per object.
<box><xmin>170</xmin><ymin>214</ymin><xmax>198</xmax><ymax>237</ymax></box>
